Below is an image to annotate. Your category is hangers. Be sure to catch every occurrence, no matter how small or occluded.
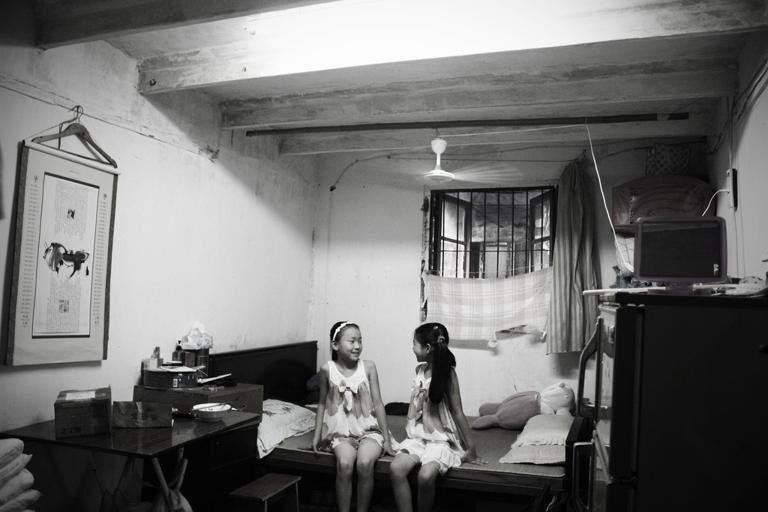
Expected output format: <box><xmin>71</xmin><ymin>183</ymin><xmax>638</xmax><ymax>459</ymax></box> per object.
<box><xmin>32</xmin><ymin>105</ymin><xmax>119</xmax><ymax>168</ymax></box>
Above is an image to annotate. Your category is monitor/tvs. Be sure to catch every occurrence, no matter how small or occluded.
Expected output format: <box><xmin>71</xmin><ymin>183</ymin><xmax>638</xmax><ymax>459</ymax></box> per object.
<box><xmin>632</xmin><ymin>215</ymin><xmax>727</xmax><ymax>297</ymax></box>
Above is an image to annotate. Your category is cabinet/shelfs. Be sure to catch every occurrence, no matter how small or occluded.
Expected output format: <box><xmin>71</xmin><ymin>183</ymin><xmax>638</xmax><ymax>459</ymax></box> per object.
<box><xmin>135</xmin><ymin>383</ymin><xmax>263</xmax><ymax>511</ymax></box>
<box><xmin>612</xmin><ymin>174</ymin><xmax>716</xmax><ymax>234</ymax></box>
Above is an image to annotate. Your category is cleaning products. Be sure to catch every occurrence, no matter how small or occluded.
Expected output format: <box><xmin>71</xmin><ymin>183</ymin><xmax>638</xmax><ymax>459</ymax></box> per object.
<box><xmin>171</xmin><ymin>339</ymin><xmax>185</xmax><ymax>365</ymax></box>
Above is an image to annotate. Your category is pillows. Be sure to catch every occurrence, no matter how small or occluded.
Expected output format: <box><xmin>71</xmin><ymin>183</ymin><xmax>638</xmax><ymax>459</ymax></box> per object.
<box><xmin>256</xmin><ymin>398</ymin><xmax>316</xmax><ymax>460</ymax></box>
<box><xmin>498</xmin><ymin>415</ymin><xmax>574</xmax><ymax>464</ymax></box>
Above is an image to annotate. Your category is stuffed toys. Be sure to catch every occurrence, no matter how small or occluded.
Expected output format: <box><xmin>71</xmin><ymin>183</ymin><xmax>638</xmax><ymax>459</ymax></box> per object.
<box><xmin>472</xmin><ymin>380</ymin><xmax>577</xmax><ymax>432</ymax></box>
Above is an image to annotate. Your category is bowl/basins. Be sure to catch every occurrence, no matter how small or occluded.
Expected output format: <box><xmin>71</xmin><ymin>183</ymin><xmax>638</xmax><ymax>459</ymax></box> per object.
<box><xmin>192</xmin><ymin>402</ymin><xmax>232</xmax><ymax>424</ymax></box>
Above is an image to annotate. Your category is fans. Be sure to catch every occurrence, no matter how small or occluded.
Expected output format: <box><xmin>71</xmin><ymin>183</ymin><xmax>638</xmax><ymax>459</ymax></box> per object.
<box><xmin>363</xmin><ymin>136</ymin><xmax>524</xmax><ymax>188</ymax></box>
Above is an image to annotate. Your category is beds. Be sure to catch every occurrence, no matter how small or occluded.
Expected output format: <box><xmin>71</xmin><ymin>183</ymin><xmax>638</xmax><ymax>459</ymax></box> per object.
<box><xmin>210</xmin><ymin>341</ymin><xmax>589</xmax><ymax>511</ymax></box>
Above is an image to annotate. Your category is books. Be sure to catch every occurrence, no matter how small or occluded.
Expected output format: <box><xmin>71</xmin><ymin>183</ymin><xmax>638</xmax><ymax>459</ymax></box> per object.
<box><xmin>581</xmin><ymin>286</ymin><xmax>671</xmax><ymax>295</ymax></box>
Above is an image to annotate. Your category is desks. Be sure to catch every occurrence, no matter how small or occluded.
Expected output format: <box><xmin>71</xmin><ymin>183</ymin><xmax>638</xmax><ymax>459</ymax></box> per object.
<box><xmin>0</xmin><ymin>411</ymin><xmax>262</xmax><ymax>512</ymax></box>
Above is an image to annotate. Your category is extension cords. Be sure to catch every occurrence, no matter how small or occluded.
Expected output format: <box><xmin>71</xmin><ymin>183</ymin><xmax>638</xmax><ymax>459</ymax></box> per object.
<box><xmin>725</xmin><ymin>167</ymin><xmax>737</xmax><ymax>209</ymax></box>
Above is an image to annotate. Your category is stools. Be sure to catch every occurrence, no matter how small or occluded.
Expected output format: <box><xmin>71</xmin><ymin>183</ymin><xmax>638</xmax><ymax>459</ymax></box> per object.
<box><xmin>229</xmin><ymin>473</ymin><xmax>302</xmax><ymax>512</ymax></box>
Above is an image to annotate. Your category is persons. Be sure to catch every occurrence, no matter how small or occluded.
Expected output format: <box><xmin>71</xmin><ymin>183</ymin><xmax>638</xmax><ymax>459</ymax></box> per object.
<box><xmin>296</xmin><ymin>320</ymin><xmax>397</xmax><ymax>511</ymax></box>
<box><xmin>388</xmin><ymin>322</ymin><xmax>489</xmax><ymax>511</ymax></box>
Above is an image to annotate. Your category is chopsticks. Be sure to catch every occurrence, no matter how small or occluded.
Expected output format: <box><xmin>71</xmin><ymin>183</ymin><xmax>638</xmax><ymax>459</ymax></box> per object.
<box><xmin>190</xmin><ymin>399</ymin><xmax>233</xmax><ymax>411</ymax></box>
